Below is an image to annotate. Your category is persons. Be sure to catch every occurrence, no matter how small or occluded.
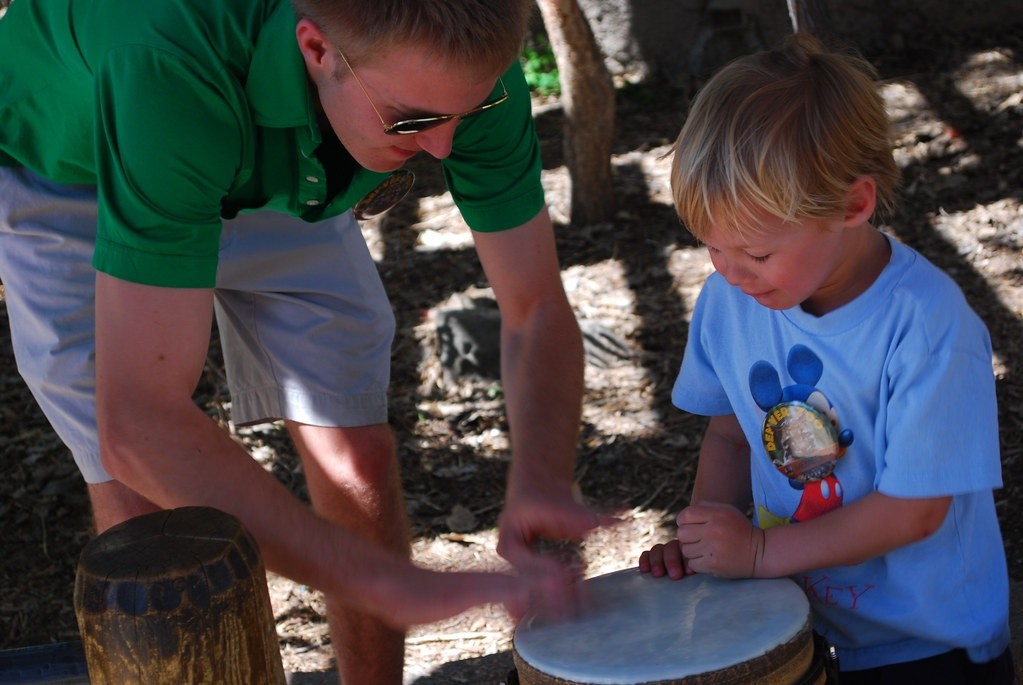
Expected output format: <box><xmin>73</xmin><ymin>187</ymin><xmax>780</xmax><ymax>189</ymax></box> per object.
<box><xmin>0</xmin><ymin>0</ymin><xmax>627</xmax><ymax>685</ymax></box>
<box><xmin>638</xmin><ymin>33</ymin><xmax>1013</xmax><ymax>685</ymax></box>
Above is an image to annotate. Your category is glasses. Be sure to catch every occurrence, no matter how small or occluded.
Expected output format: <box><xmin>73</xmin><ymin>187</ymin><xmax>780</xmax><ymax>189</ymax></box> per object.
<box><xmin>336</xmin><ymin>44</ymin><xmax>510</xmax><ymax>136</ymax></box>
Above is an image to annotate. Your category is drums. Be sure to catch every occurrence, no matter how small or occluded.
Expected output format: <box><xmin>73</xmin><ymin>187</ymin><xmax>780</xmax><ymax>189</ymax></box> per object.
<box><xmin>512</xmin><ymin>565</ymin><xmax>840</xmax><ymax>685</ymax></box>
